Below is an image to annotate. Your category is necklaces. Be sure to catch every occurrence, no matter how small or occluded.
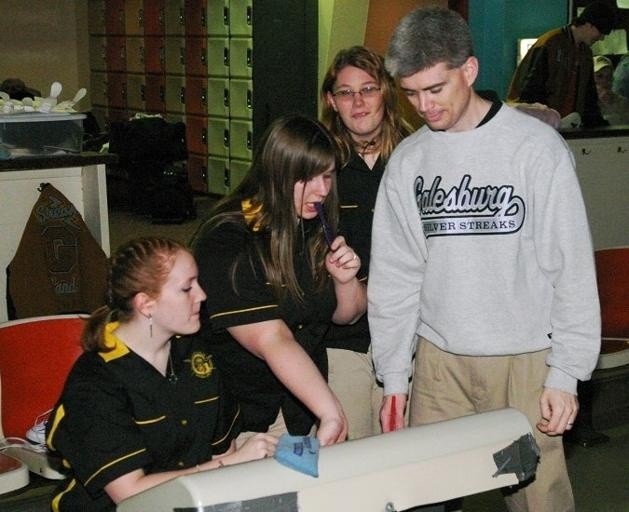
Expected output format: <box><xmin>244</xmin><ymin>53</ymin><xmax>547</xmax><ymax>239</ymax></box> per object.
<box><xmin>362</xmin><ymin>139</ymin><xmax>379</xmax><ymax>148</ymax></box>
<box><xmin>115</xmin><ymin>328</ymin><xmax>179</xmax><ymax>383</ymax></box>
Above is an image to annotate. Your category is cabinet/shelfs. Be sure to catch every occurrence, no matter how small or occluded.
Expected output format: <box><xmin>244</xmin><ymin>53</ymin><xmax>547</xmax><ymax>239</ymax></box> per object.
<box><xmin>82</xmin><ymin>1</ymin><xmax>318</xmax><ymax>196</ymax></box>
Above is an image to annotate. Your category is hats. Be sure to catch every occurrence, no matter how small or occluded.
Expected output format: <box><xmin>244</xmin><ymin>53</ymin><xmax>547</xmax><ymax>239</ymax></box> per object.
<box><xmin>580</xmin><ymin>2</ymin><xmax>616</xmax><ymax>35</ymax></box>
<box><xmin>593</xmin><ymin>55</ymin><xmax>610</xmax><ymax>72</ymax></box>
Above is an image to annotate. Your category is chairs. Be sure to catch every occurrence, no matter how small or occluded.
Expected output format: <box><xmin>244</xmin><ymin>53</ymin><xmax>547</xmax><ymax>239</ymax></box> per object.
<box><xmin>0</xmin><ymin>314</ymin><xmax>113</xmax><ymax>481</ymax></box>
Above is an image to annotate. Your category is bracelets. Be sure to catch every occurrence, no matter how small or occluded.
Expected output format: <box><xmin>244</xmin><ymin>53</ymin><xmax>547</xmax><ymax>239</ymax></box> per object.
<box><xmin>194</xmin><ymin>463</ymin><xmax>202</xmax><ymax>472</ymax></box>
<box><xmin>217</xmin><ymin>457</ymin><xmax>225</xmax><ymax>469</ymax></box>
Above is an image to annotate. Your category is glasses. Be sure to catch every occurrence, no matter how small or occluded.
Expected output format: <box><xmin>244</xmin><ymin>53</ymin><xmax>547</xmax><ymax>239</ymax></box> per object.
<box><xmin>332</xmin><ymin>86</ymin><xmax>381</xmax><ymax>100</ymax></box>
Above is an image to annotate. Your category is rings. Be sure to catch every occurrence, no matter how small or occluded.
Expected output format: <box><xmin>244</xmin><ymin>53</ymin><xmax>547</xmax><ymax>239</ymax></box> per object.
<box><xmin>567</xmin><ymin>421</ymin><xmax>574</xmax><ymax>426</ymax></box>
<box><xmin>352</xmin><ymin>251</ymin><xmax>357</xmax><ymax>260</ymax></box>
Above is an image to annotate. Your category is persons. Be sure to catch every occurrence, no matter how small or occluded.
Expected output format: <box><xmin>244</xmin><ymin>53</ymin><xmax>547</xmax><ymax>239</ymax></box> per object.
<box><xmin>317</xmin><ymin>43</ymin><xmax>418</xmax><ymax>442</ymax></box>
<box><xmin>43</xmin><ymin>235</ymin><xmax>281</xmax><ymax>512</ymax></box>
<box><xmin>186</xmin><ymin>111</ymin><xmax>369</xmax><ymax>461</ymax></box>
<box><xmin>592</xmin><ymin>54</ymin><xmax>629</xmax><ymax>127</ymax></box>
<box><xmin>366</xmin><ymin>0</ymin><xmax>604</xmax><ymax>511</ymax></box>
<box><xmin>506</xmin><ymin>0</ymin><xmax>622</xmax><ymax>128</ymax></box>
